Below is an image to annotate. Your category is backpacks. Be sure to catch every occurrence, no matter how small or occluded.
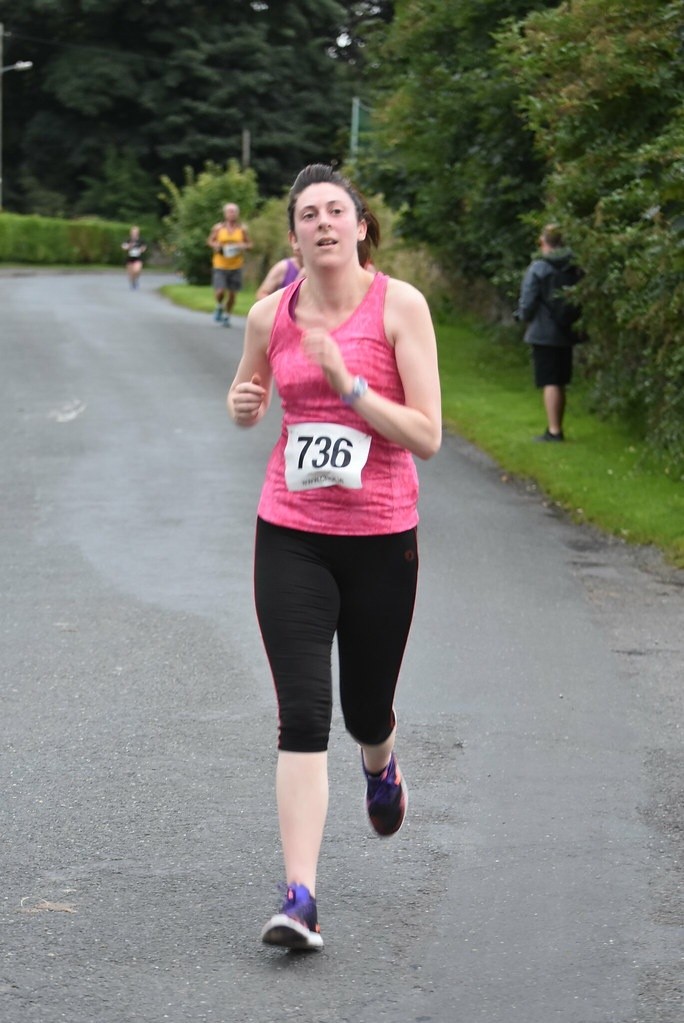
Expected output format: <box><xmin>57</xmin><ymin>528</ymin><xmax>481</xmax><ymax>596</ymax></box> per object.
<box><xmin>531</xmin><ymin>254</ymin><xmax>586</xmax><ymax>328</ymax></box>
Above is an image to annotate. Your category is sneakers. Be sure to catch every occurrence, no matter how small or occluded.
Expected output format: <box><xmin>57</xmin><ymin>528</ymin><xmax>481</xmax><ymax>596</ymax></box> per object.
<box><xmin>360</xmin><ymin>744</ymin><xmax>408</xmax><ymax>838</ymax></box>
<box><xmin>261</xmin><ymin>880</ymin><xmax>326</xmax><ymax>951</ymax></box>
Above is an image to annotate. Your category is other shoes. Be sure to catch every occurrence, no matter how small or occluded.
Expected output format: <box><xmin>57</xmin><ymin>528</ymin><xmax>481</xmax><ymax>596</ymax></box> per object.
<box><xmin>533</xmin><ymin>427</ymin><xmax>566</xmax><ymax>443</ymax></box>
<box><xmin>214</xmin><ymin>304</ymin><xmax>233</xmax><ymax>330</ymax></box>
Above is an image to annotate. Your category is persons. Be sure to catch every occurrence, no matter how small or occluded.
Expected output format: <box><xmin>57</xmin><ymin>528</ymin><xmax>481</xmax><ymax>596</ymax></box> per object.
<box><xmin>511</xmin><ymin>223</ymin><xmax>586</xmax><ymax>443</ymax></box>
<box><xmin>206</xmin><ymin>203</ymin><xmax>255</xmax><ymax>326</ymax></box>
<box><xmin>224</xmin><ymin>163</ymin><xmax>442</xmax><ymax>950</ymax></box>
<box><xmin>255</xmin><ymin>225</ymin><xmax>305</xmax><ymax>300</ymax></box>
<box><xmin>121</xmin><ymin>226</ymin><xmax>147</xmax><ymax>290</ymax></box>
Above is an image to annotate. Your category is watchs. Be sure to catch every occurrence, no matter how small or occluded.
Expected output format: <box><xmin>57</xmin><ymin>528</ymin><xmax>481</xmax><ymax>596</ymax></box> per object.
<box><xmin>339</xmin><ymin>373</ymin><xmax>368</xmax><ymax>405</ymax></box>
